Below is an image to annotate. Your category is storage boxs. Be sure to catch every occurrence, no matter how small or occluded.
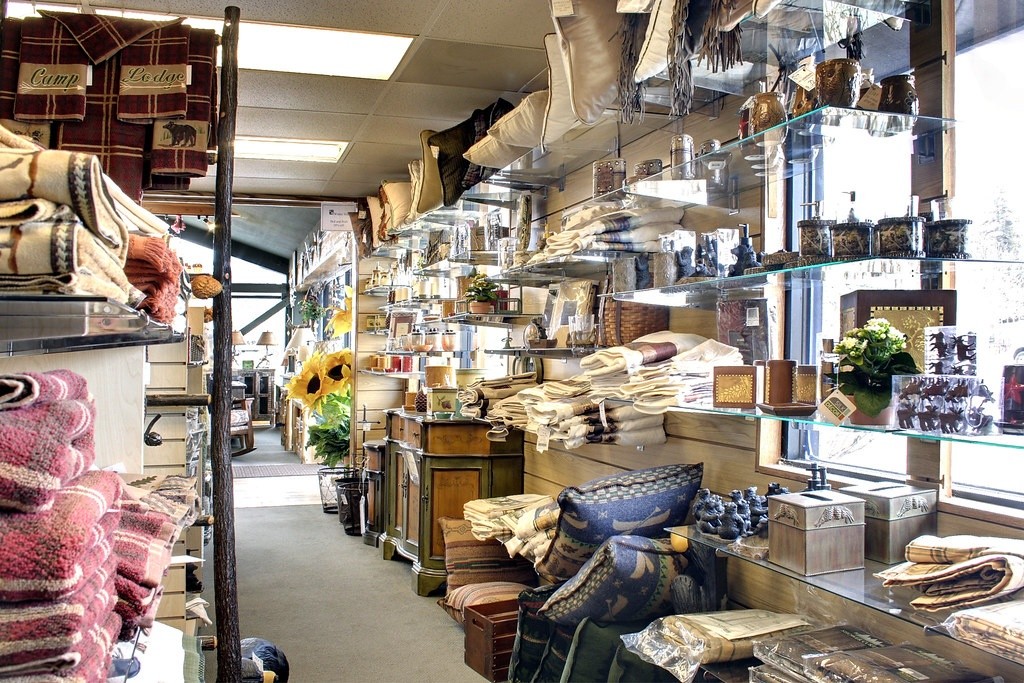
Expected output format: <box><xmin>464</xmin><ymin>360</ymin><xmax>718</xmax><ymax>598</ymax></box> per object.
<box><xmin>701</xmin><ymin>227</ymin><xmax>741</xmax><ymax>277</ymax></box>
<box><xmin>756</xmin><ymin>359</ymin><xmax>818</xmax><ymax>418</ymax></box>
<box><xmin>766</xmin><ymin>489</ymin><xmax>865</xmax><ymax>576</ymax></box>
<box><xmin>660</xmin><ymin>230</ymin><xmax>696</xmax><ymax>266</ymax></box>
<box><xmin>838</xmin><ymin>479</ymin><xmax>939</xmax><ymax>565</ymax></box>
<box><xmin>761</xmin><ymin>251</ymin><xmax>800</xmax><ymax>267</ymax></box>
<box><xmin>838</xmin><ymin>288</ymin><xmax>958</xmax><ymax>373</ymax></box>
<box><xmin>798</xmin><ymin>366</ymin><xmax>817</xmax><ymax>405</ymax></box>
<box><xmin>469</xmin><ymin>226</ymin><xmax>485</xmax><ymax>250</ymax></box>
<box><xmin>713</xmin><ymin>365</ymin><xmax>757</xmax><ymax>410</ymax></box>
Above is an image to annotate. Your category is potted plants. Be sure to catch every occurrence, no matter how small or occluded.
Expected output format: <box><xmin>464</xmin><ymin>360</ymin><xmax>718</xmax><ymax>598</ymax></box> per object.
<box><xmin>462</xmin><ymin>273</ymin><xmax>501</xmax><ymax>314</ymax></box>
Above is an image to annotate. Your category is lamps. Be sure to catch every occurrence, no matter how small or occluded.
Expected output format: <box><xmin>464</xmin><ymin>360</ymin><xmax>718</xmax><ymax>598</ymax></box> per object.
<box><xmin>286</xmin><ymin>327</ymin><xmax>318</xmax><ymax>367</ymax></box>
<box><xmin>256</xmin><ymin>330</ymin><xmax>279</xmax><ymax>368</ymax></box>
<box><xmin>232</xmin><ymin>329</ymin><xmax>247</xmax><ymax>367</ymax></box>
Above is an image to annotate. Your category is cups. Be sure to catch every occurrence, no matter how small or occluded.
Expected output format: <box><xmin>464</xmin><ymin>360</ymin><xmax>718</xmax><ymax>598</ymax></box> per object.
<box><xmin>369</xmin><ymin>355</ymin><xmax>413</xmax><ymax>372</ymax></box>
<box><xmin>796</xmin><ymin>216</ymin><xmax>973</xmax><ymax>260</ymax></box>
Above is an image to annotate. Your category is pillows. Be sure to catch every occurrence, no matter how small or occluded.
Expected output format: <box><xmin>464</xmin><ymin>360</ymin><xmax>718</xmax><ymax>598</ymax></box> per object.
<box><xmin>403</xmin><ymin>158</ymin><xmax>421</xmax><ymax>225</ymax></box>
<box><xmin>378</xmin><ymin>186</ymin><xmax>394</xmax><ymax>241</ymax></box>
<box><xmin>463</xmin><ymin>134</ymin><xmax>532</xmax><ymax>168</ymax></box>
<box><xmin>608</xmin><ymin>645</ymin><xmax>682</xmax><ymax>683</ymax></box>
<box><xmin>436</xmin><ymin>581</ymin><xmax>534</xmax><ymax>627</ymax></box>
<box><xmin>416</xmin><ymin>129</ymin><xmax>444</xmax><ymax>214</ymax></box>
<box><xmin>426</xmin><ymin>97</ymin><xmax>514</xmax><ymax>207</ymax></box>
<box><xmin>504</xmin><ymin>586</ymin><xmax>552</xmax><ymax>683</ymax></box>
<box><xmin>381</xmin><ymin>178</ymin><xmax>411</xmax><ymax>230</ymax></box>
<box><xmin>751</xmin><ymin>0</ymin><xmax>908</xmax><ymax>32</ymax></box>
<box><xmin>486</xmin><ymin>89</ymin><xmax>549</xmax><ymax>151</ymax></box>
<box><xmin>534</xmin><ymin>460</ymin><xmax>704</xmax><ymax>581</ymax></box>
<box><xmin>539</xmin><ymin>31</ymin><xmax>579</xmax><ymax>154</ymax></box>
<box><xmin>439</xmin><ymin>516</ymin><xmax>535</xmax><ymax>594</ymax></box>
<box><xmin>538</xmin><ymin>534</ymin><xmax>709</xmax><ymax>634</ymax></box>
<box><xmin>365</xmin><ymin>195</ymin><xmax>386</xmax><ymax>250</ymax></box>
<box><xmin>548</xmin><ymin>0</ymin><xmax>625</xmax><ymax>127</ymax></box>
<box><xmin>559</xmin><ymin>616</ymin><xmax>644</xmax><ymax>683</ymax></box>
<box><xmin>633</xmin><ymin>0</ymin><xmax>677</xmax><ymax>82</ymax></box>
<box><xmin>530</xmin><ymin>626</ymin><xmax>572</xmax><ymax>683</ymax></box>
<box><xmin>347</xmin><ymin>211</ymin><xmax>368</xmax><ymax>261</ymax></box>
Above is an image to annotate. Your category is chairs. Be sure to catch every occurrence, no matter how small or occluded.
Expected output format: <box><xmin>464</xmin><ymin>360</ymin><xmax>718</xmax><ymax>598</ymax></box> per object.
<box><xmin>231</xmin><ymin>397</ymin><xmax>259</xmax><ymax>458</ymax></box>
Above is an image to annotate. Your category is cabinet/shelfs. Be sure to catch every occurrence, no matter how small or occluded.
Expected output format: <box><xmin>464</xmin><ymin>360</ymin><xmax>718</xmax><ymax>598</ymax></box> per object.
<box><xmin>0</xmin><ymin>0</ymin><xmax>1024</xmax><ymax>683</ymax></box>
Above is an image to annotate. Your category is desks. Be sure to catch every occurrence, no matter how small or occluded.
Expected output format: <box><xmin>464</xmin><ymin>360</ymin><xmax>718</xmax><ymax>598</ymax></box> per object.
<box><xmin>231</xmin><ymin>380</ymin><xmax>248</xmax><ymax>449</ymax></box>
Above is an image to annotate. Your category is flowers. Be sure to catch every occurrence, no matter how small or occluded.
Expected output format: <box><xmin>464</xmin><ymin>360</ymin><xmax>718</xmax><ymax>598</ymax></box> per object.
<box><xmin>280</xmin><ymin>347</ymin><xmax>354</xmax><ymax>465</ymax></box>
<box><xmin>323</xmin><ymin>284</ymin><xmax>352</xmax><ymax>339</ymax></box>
<box><xmin>823</xmin><ymin>316</ymin><xmax>923</xmax><ymax>415</ymax></box>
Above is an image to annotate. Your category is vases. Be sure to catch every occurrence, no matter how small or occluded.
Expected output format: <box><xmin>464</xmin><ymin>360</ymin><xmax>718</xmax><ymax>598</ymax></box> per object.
<box><xmin>844</xmin><ymin>394</ymin><xmax>896</xmax><ymax>426</ymax></box>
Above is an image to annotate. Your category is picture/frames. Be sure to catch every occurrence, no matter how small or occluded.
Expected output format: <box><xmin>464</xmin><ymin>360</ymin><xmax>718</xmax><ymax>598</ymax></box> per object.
<box><xmin>391</xmin><ymin>312</ymin><xmax>418</xmax><ymax>339</ymax></box>
